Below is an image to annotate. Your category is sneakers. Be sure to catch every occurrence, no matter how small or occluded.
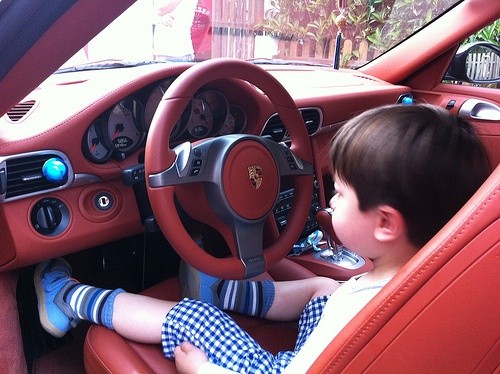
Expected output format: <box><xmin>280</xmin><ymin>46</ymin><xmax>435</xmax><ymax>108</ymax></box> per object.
<box><xmin>34</xmin><ymin>257</ymin><xmax>81</xmax><ymax>337</ymax></box>
<box><xmin>178</xmin><ymin>231</ymin><xmax>226</xmax><ymax>311</ymax></box>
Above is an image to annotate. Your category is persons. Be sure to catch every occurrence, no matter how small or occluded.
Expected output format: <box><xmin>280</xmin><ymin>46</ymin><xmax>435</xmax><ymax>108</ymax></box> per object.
<box><xmin>31</xmin><ymin>102</ymin><xmax>489</xmax><ymax>373</ymax></box>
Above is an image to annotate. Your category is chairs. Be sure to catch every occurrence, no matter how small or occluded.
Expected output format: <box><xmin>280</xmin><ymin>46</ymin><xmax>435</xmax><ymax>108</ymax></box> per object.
<box><xmin>83</xmin><ymin>161</ymin><xmax>500</xmax><ymax>374</ymax></box>
